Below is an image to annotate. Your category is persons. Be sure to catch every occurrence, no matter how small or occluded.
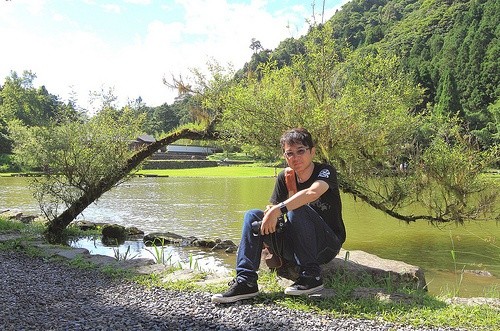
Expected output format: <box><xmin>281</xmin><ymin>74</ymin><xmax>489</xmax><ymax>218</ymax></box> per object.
<box><xmin>210</xmin><ymin>128</ymin><xmax>346</xmax><ymax>303</ymax></box>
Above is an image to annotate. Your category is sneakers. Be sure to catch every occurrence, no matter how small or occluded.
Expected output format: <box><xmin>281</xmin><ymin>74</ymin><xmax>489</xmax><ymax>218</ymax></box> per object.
<box><xmin>211</xmin><ymin>278</ymin><xmax>258</xmax><ymax>303</ymax></box>
<box><xmin>284</xmin><ymin>274</ymin><xmax>324</xmax><ymax>296</ymax></box>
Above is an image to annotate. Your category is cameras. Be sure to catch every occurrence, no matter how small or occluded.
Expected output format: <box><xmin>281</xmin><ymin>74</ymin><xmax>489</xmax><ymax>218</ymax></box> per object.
<box><xmin>251</xmin><ymin>215</ymin><xmax>287</xmax><ymax>235</ymax></box>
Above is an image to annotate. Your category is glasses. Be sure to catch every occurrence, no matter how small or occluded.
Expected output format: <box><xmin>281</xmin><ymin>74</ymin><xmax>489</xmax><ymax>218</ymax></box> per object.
<box><xmin>284</xmin><ymin>147</ymin><xmax>311</xmax><ymax>159</ymax></box>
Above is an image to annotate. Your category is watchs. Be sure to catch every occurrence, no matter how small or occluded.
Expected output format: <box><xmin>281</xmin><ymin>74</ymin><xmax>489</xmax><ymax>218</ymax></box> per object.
<box><xmin>277</xmin><ymin>202</ymin><xmax>289</xmax><ymax>215</ymax></box>
